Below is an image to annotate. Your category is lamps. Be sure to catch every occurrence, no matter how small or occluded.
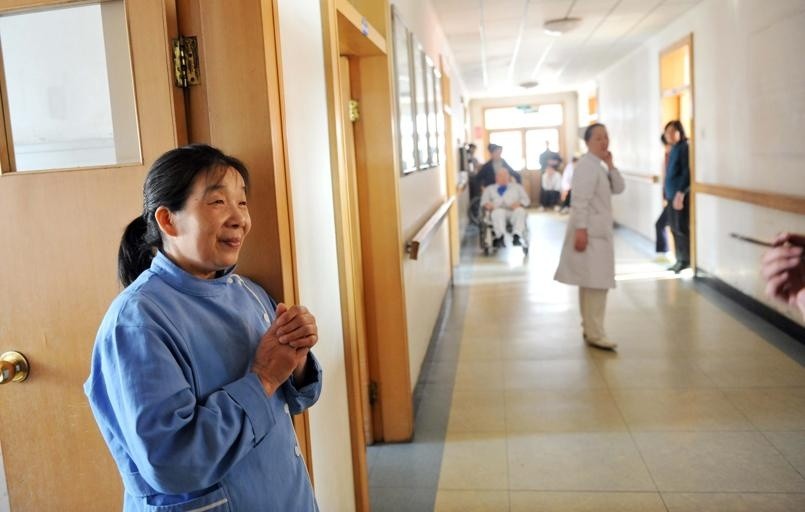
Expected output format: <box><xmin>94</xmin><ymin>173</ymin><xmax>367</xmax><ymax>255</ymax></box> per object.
<box><xmin>544</xmin><ymin>17</ymin><xmax>583</xmax><ymax>33</ymax></box>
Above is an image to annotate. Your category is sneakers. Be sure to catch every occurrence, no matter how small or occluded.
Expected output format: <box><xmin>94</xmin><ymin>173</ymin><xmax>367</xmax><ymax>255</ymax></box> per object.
<box><xmin>583</xmin><ymin>333</ymin><xmax>619</xmax><ymax>350</ymax></box>
<box><xmin>665</xmin><ymin>259</ymin><xmax>693</xmax><ymax>274</ymax></box>
<box><xmin>494</xmin><ymin>235</ymin><xmax>521</xmax><ymax>248</ymax></box>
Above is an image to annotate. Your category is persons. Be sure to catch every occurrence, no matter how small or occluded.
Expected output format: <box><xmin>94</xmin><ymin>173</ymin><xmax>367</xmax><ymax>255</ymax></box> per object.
<box><xmin>465</xmin><ymin>140</ymin><xmax>530</xmax><ymax>250</ymax></box>
<box><xmin>539</xmin><ymin>139</ymin><xmax>580</xmax><ymax>215</ymax></box>
<box><xmin>654</xmin><ymin>133</ymin><xmax>675</xmax><ymax>263</ymax></box>
<box><xmin>552</xmin><ymin>122</ymin><xmax>625</xmax><ymax>349</ymax></box>
<box><xmin>662</xmin><ymin>122</ymin><xmax>690</xmax><ymax>275</ymax></box>
<box><xmin>760</xmin><ymin>230</ymin><xmax>804</xmax><ymax>318</ymax></box>
<box><xmin>82</xmin><ymin>142</ymin><xmax>323</xmax><ymax>512</ymax></box>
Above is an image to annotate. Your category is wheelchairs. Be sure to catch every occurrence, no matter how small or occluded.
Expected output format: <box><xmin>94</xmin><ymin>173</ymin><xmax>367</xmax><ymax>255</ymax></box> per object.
<box><xmin>478</xmin><ymin>206</ymin><xmax>530</xmax><ymax>256</ymax></box>
